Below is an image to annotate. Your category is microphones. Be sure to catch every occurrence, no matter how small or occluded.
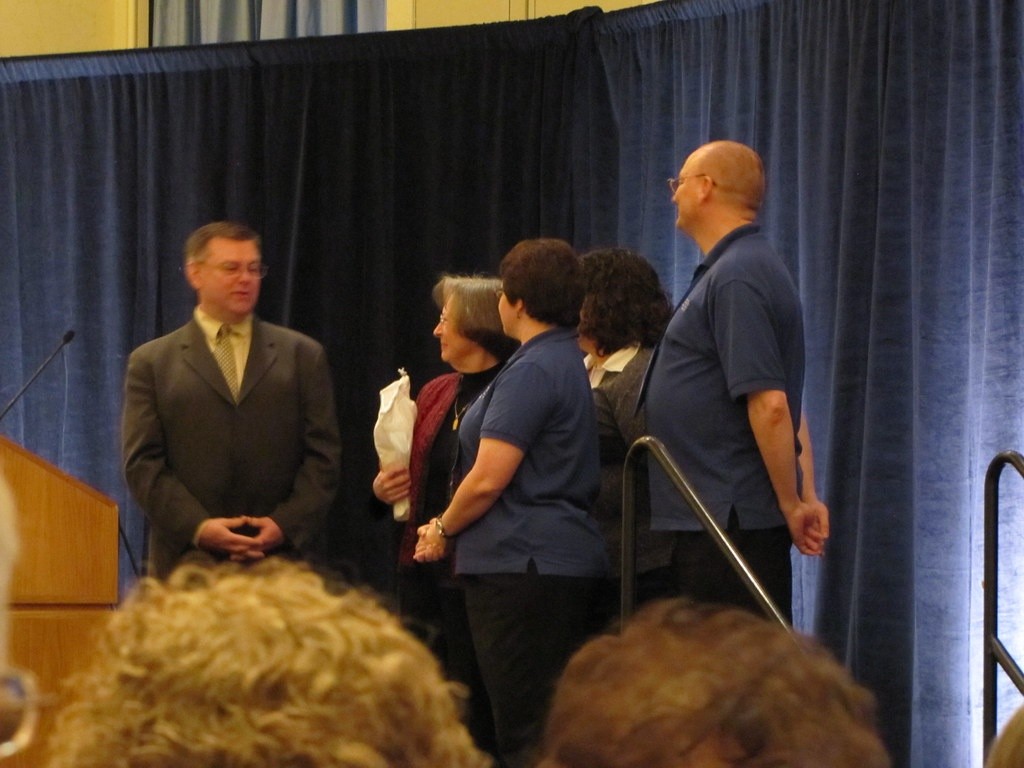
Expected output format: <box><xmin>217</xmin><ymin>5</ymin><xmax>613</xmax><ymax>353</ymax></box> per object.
<box><xmin>0</xmin><ymin>329</ymin><xmax>75</xmax><ymax>421</ymax></box>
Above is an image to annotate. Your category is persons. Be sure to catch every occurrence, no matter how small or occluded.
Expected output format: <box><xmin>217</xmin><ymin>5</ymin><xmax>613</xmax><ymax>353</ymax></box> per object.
<box><xmin>644</xmin><ymin>140</ymin><xmax>831</xmax><ymax>626</ymax></box>
<box><xmin>46</xmin><ymin>560</ymin><xmax>493</xmax><ymax>768</ymax></box>
<box><xmin>119</xmin><ymin>222</ymin><xmax>340</xmax><ymax>581</ymax></box>
<box><xmin>537</xmin><ymin>597</ymin><xmax>892</xmax><ymax>767</ymax></box>
<box><xmin>371</xmin><ymin>274</ymin><xmax>522</xmax><ymax>755</ymax></box>
<box><xmin>572</xmin><ymin>247</ymin><xmax>675</xmax><ymax>634</ymax></box>
<box><xmin>414</xmin><ymin>240</ymin><xmax>607</xmax><ymax>768</ymax></box>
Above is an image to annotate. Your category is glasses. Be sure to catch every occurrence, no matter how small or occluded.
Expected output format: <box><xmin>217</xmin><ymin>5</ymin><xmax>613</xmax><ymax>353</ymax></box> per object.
<box><xmin>668</xmin><ymin>173</ymin><xmax>717</xmax><ymax>194</ymax></box>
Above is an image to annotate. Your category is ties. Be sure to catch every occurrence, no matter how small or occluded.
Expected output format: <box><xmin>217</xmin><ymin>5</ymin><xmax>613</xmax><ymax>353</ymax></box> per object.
<box><xmin>214</xmin><ymin>323</ymin><xmax>239</xmax><ymax>403</ymax></box>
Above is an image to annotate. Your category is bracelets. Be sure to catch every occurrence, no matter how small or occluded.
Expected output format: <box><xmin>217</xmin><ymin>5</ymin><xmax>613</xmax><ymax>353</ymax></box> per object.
<box><xmin>435</xmin><ymin>514</ymin><xmax>448</xmax><ymax>539</ymax></box>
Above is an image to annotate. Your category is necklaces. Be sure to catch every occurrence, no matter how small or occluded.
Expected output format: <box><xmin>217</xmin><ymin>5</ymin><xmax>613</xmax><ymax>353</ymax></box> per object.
<box><xmin>450</xmin><ymin>399</ymin><xmax>470</xmax><ymax>430</ymax></box>
